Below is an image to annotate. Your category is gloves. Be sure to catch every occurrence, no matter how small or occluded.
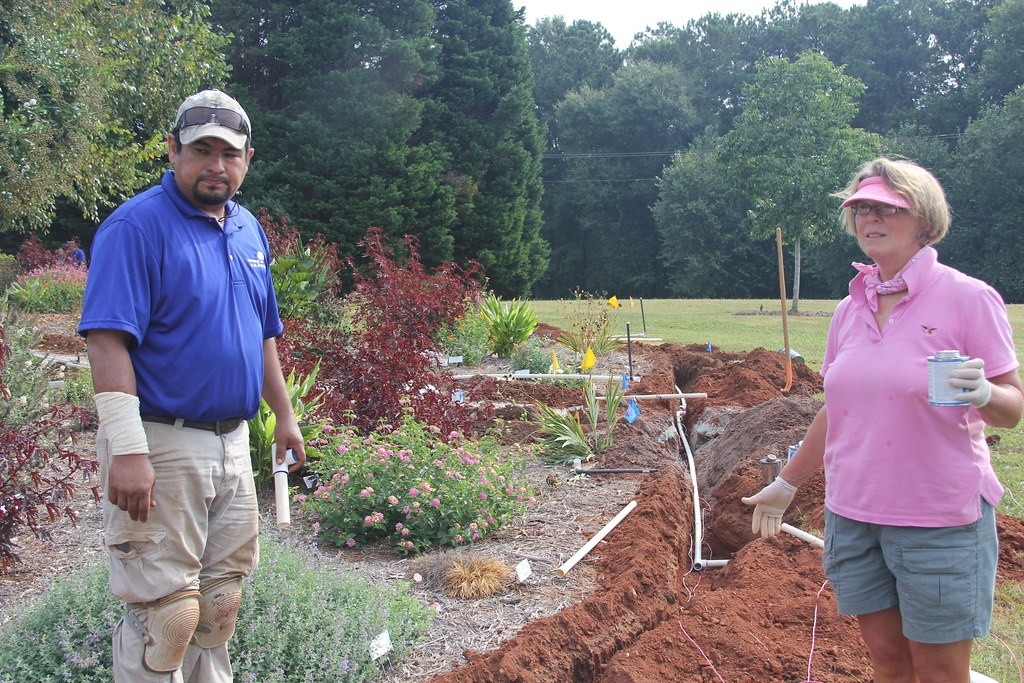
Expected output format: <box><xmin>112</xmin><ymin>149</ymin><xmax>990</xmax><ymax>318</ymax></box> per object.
<box><xmin>927</xmin><ymin>353</ymin><xmax>992</xmax><ymax>408</ymax></box>
<box><xmin>741</xmin><ymin>476</ymin><xmax>797</xmax><ymax>538</ymax></box>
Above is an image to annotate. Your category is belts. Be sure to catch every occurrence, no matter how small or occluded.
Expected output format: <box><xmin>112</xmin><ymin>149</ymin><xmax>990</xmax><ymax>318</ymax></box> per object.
<box><xmin>138</xmin><ymin>409</ymin><xmax>243</xmax><ymax>435</ymax></box>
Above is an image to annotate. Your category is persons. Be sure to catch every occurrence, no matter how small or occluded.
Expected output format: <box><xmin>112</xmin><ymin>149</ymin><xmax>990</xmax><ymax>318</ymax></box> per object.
<box><xmin>66</xmin><ymin>236</ymin><xmax>86</xmax><ymax>266</ymax></box>
<box><xmin>742</xmin><ymin>154</ymin><xmax>1024</xmax><ymax>683</ymax></box>
<box><xmin>77</xmin><ymin>88</ymin><xmax>307</xmax><ymax>683</ymax></box>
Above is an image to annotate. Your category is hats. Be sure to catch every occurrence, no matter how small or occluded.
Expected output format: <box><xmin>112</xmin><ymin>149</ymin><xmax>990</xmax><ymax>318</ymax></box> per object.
<box><xmin>842</xmin><ymin>176</ymin><xmax>912</xmax><ymax>208</ymax></box>
<box><xmin>172</xmin><ymin>89</ymin><xmax>251</xmax><ymax>150</ymax></box>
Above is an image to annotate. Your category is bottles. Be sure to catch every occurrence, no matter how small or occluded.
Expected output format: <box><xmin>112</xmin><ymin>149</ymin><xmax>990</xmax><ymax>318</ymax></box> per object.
<box><xmin>926</xmin><ymin>350</ymin><xmax>970</xmax><ymax>407</ymax></box>
<box><xmin>761</xmin><ymin>455</ymin><xmax>781</xmax><ymax>485</ymax></box>
<box><xmin>788</xmin><ymin>441</ymin><xmax>802</xmax><ymax>462</ymax></box>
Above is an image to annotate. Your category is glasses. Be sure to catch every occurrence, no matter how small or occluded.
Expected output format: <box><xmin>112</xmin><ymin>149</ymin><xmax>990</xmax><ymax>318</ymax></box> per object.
<box><xmin>173</xmin><ymin>106</ymin><xmax>251</xmax><ymax>141</ymax></box>
<box><xmin>851</xmin><ymin>201</ymin><xmax>903</xmax><ymax>216</ymax></box>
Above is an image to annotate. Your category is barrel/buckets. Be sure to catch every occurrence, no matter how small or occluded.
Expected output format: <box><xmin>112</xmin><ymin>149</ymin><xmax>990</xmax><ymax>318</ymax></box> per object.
<box><xmin>778</xmin><ymin>348</ymin><xmax>804</xmax><ymax>363</ymax></box>
